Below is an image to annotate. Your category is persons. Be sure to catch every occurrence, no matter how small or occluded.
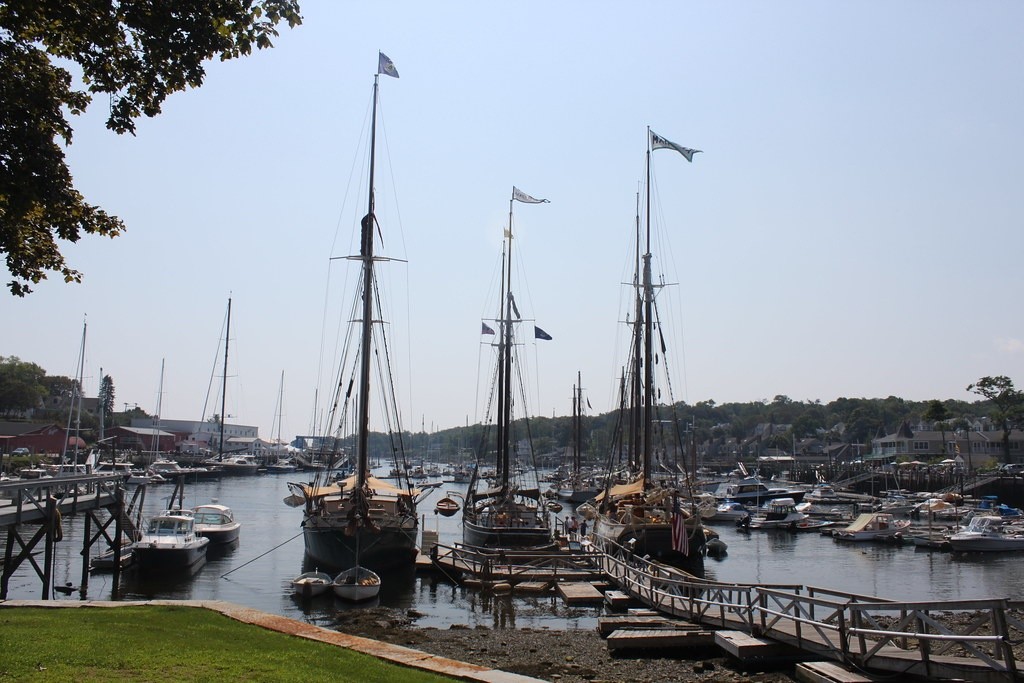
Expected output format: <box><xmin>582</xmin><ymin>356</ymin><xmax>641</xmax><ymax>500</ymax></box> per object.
<box><xmin>912</xmin><ymin>465</ymin><xmax>916</xmax><ymax>470</ymax></box>
<box><xmin>565</xmin><ymin>516</ymin><xmax>579</xmax><ymax>534</ymax></box>
<box><xmin>579</xmin><ymin>520</ymin><xmax>589</xmax><ymax>537</ymax></box>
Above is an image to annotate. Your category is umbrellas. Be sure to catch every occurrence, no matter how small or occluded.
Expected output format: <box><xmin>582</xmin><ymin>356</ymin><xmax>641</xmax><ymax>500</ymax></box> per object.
<box><xmin>890</xmin><ymin>461</ymin><xmax>927</xmax><ymax>465</ymax></box>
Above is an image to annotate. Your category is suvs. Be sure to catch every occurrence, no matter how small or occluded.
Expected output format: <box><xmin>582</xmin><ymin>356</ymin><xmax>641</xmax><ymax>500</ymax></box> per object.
<box><xmin>12</xmin><ymin>448</ymin><xmax>29</xmax><ymax>457</ymax></box>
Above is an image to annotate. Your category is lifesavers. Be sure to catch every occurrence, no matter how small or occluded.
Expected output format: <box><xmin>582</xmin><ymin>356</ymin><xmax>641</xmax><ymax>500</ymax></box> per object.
<box><xmin>654</xmin><ymin>517</ymin><xmax>663</xmax><ymax>523</ymax></box>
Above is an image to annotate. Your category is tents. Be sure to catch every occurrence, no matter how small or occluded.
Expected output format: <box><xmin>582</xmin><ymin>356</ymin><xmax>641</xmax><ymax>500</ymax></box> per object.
<box><xmin>942</xmin><ymin>459</ymin><xmax>955</xmax><ymax>466</ymax></box>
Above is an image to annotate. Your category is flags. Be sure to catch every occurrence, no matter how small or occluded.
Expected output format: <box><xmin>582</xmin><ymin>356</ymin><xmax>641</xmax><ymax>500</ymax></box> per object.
<box><xmin>482</xmin><ymin>323</ymin><xmax>494</xmax><ymax>334</ymax></box>
<box><xmin>650</xmin><ymin>132</ymin><xmax>703</xmax><ymax>161</ymax></box>
<box><xmin>535</xmin><ymin>326</ymin><xmax>552</xmax><ymax>340</ymax></box>
<box><xmin>378</xmin><ymin>52</ymin><xmax>400</xmax><ymax>78</ymax></box>
<box><xmin>672</xmin><ymin>509</ymin><xmax>688</xmax><ymax>556</ymax></box>
<box><xmin>505</xmin><ymin>229</ymin><xmax>514</xmax><ymax>239</ymax></box>
<box><xmin>513</xmin><ymin>187</ymin><xmax>550</xmax><ymax>203</ymax></box>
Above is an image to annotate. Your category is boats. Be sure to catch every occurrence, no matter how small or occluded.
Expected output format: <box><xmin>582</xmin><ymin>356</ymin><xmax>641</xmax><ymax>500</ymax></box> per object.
<box><xmin>332</xmin><ymin>565</ymin><xmax>380</xmax><ymax>600</ymax></box>
<box><xmin>484</xmin><ymin>543</ymin><xmax>558</xmax><ymax>559</ymax></box>
<box><xmin>546</xmin><ymin>501</ymin><xmax>562</xmax><ymax>513</ymax></box>
<box><xmin>292</xmin><ymin>572</ymin><xmax>332</xmax><ymax>601</ymax></box>
<box><xmin>538</xmin><ymin>371</ymin><xmax>1024</xmax><ymax>551</ymax></box>
<box><xmin>284</xmin><ymin>494</ymin><xmax>305</xmax><ymax>508</ymax></box>
<box><xmin>131</xmin><ymin>504</ymin><xmax>210</xmax><ymax>568</ymax></box>
<box><xmin>437</xmin><ymin>498</ymin><xmax>459</xmax><ymax>517</ymax></box>
<box><xmin>173</xmin><ymin>498</ymin><xmax>240</xmax><ymax>546</ymax></box>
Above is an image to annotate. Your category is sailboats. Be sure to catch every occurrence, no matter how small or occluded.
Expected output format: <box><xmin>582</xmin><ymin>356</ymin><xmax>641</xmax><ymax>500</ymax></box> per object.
<box><xmin>590</xmin><ymin>125</ymin><xmax>708</xmax><ymax>565</ymax></box>
<box><xmin>0</xmin><ymin>291</ymin><xmax>539</xmax><ymax>508</ymax></box>
<box><xmin>462</xmin><ymin>185</ymin><xmax>552</xmax><ymax>558</ymax></box>
